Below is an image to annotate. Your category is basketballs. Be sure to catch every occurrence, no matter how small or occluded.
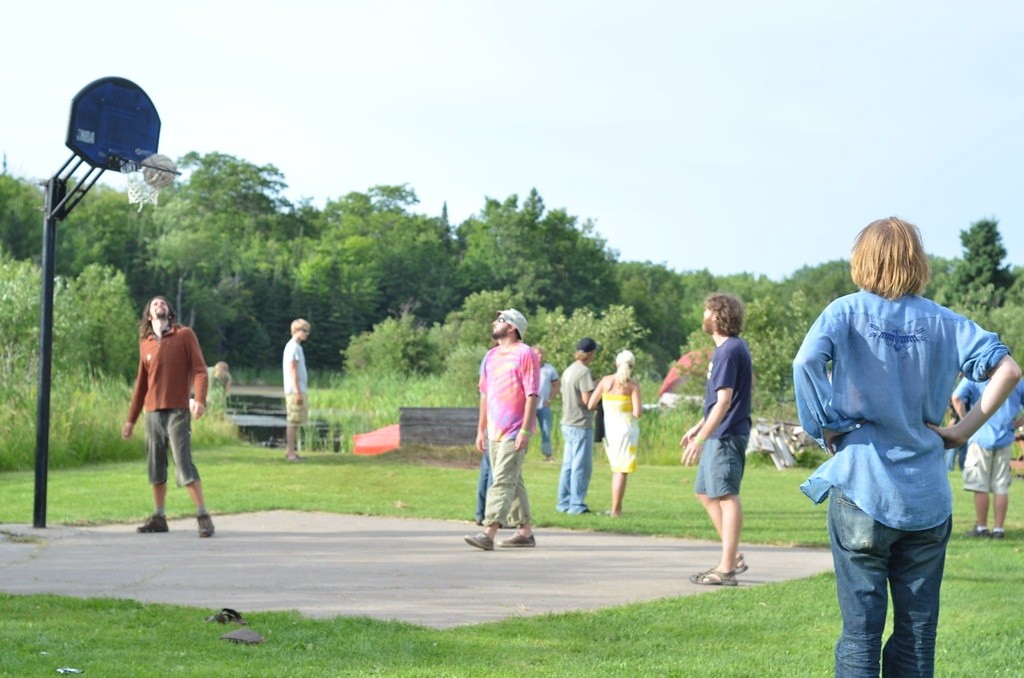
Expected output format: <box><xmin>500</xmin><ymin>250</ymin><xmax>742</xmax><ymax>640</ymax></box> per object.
<box><xmin>143</xmin><ymin>154</ymin><xmax>177</xmax><ymax>188</ymax></box>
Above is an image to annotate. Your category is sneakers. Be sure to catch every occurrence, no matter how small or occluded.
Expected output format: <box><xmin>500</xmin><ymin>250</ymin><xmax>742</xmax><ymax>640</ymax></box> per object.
<box><xmin>496</xmin><ymin>531</ymin><xmax>536</xmax><ymax>547</ymax></box>
<box><xmin>464</xmin><ymin>530</ymin><xmax>495</xmax><ymax>550</ymax></box>
<box><xmin>197</xmin><ymin>515</ymin><xmax>215</xmax><ymax>538</ymax></box>
<box><xmin>136</xmin><ymin>513</ymin><xmax>169</xmax><ymax>534</ymax></box>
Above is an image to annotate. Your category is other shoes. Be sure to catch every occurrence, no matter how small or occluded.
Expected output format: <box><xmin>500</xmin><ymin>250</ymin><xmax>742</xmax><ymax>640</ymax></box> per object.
<box><xmin>285</xmin><ymin>452</ymin><xmax>300</xmax><ymax>463</ymax></box>
<box><xmin>476</xmin><ymin>518</ymin><xmax>486</xmax><ymax>525</ymax></box>
<box><xmin>964</xmin><ymin>525</ymin><xmax>990</xmax><ymax>537</ymax></box>
<box><xmin>580</xmin><ymin>509</ymin><xmax>592</xmax><ymax>515</ymax></box>
<box><xmin>989</xmin><ymin>531</ymin><xmax>1005</xmax><ymax>539</ymax></box>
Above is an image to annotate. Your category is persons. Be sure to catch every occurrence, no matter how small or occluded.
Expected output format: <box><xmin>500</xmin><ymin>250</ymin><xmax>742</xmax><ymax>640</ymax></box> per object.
<box><xmin>679</xmin><ymin>294</ymin><xmax>754</xmax><ymax>587</ymax></box>
<box><xmin>793</xmin><ymin>214</ymin><xmax>1021</xmax><ymax>678</ymax></box>
<box><xmin>123</xmin><ymin>295</ymin><xmax>216</xmax><ymax>538</ymax></box>
<box><xmin>281</xmin><ymin>318</ymin><xmax>312</xmax><ymax>460</ymax></box>
<box><xmin>525</xmin><ymin>343</ymin><xmax>561</xmax><ymax>465</ymax></box>
<box><xmin>464</xmin><ymin>308</ymin><xmax>539</xmax><ymax>550</ymax></box>
<box><xmin>951</xmin><ymin>333</ymin><xmax>1024</xmax><ymax>538</ymax></box>
<box><xmin>587</xmin><ymin>350</ymin><xmax>641</xmax><ymax>518</ymax></box>
<box><xmin>945</xmin><ymin>393</ymin><xmax>971</xmax><ymax>472</ymax></box>
<box><xmin>208</xmin><ymin>360</ymin><xmax>233</xmax><ymax>410</ymax></box>
<box><xmin>556</xmin><ymin>336</ymin><xmax>599</xmax><ymax>514</ymax></box>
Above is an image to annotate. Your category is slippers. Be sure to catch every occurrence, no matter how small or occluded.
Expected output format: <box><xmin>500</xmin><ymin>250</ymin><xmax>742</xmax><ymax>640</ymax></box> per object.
<box><xmin>220</xmin><ymin>628</ymin><xmax>261</xmax><ymax>644</ymax></box>
<box><xmin>205</xmin><ymin>608</ymin><xmax>249</xmax><ymax>624</ymax></box>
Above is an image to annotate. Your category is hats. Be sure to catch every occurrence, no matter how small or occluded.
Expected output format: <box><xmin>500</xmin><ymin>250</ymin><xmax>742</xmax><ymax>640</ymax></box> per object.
<box><xmin>575</xmin><ymin>337</ymin><xmax>601</xmax><ymax>353</ymax></box>
<box><xmin>496</xmin><ymin>307</ymin><xmax>528</xmax><ymax>340</ymax></box>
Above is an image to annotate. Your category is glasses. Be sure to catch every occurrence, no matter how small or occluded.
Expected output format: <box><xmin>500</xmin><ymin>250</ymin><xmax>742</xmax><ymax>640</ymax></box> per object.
<box><xmin>496</xmin><ymin>317</ymin><xmax>506</xmax><ymax>323</ymax></box>
<box><xmin>300</xmin><ymin>328</ymin><xmax>311</xmax><ymax>333</ymax></box>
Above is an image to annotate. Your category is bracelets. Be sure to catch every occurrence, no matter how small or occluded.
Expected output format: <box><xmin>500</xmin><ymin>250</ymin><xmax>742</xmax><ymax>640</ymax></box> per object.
<box><xmin>694</xmin><ymin>437</ymin><xmax>704</xmax><ymax>444</ymax></box>
<box><xmin>547</xmin><ymin>399</ymin><xmax>551</xmax><ymax>403</ymax></box>
<box><xmin>520</xmin><ymin>428</ymin><xmax>532</xmax><ymax>437</ymax></box>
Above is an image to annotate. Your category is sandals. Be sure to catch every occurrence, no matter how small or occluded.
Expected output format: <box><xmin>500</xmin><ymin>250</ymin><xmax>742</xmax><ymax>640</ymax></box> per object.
<box><xmin>688</xmin><ymin>566</ymin><xmax>739</xmax><ymax>586</ymax></box>
<box><xmin>734</xmin><ymin>556</ymin><xmax>749</xmax><ymax>574</ymax></box>
